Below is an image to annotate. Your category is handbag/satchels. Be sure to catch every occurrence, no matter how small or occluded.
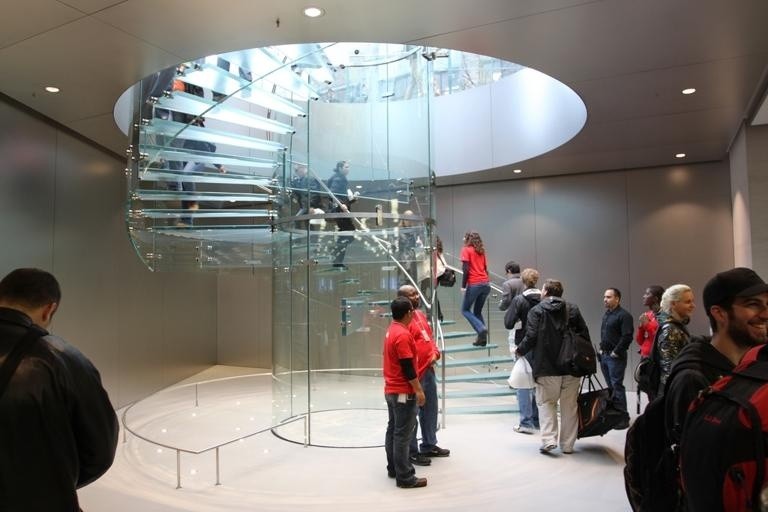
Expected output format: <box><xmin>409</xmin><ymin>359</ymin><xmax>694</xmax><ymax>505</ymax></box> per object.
<box><xmin>557</xmin><ymin>330</ymin><xmax>596</xmax><ymax>377</ymax></box>
<box><xmin>578</xmin><ymin>387</ymin><xmax>622</xmax><ymax>438</ymax></box>
<box><xmin>440</xmin><ymin>269</ymin><xmax>455</xmax><ymax>287</ymax></box>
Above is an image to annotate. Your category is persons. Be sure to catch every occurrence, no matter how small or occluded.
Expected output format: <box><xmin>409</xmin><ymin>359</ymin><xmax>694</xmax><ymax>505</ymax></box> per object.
<box><xmin>392</xmin><ymin>210</ymin><xmax>418</xmax><ymax>289</ymax></box>
<box><xmin>383</xmin><ymin>295</ymin><xmax>428</xmax><ymax>488</ymax></box>
<box><xmin>291</xmin><ymin>165</ymin><xmax>325</xmax><ymax>230</ymax></box>
<box><xmin>397</xmin><ymin>285</ymin><xmax>449</xmax><ymax>466</ymax></box>
<box><xmin>0</xmin><ymin>265</ymin><xmax>118</xmax><ymax>512</ymax></box>
<box><xmin>598</xmin><ymin>287</ymin><xmax>634</xmax><ymax>430</ymax></box>
<box><xmin>497</xmin><ymin>260</ymin><xmax>525</xmax><ymax>312</ymax></box>
<box><xmin>327</xmin><ymin>160</ymin><xmax>360</xmax><ymax>270</ymax></box>
<box><xmin>635</xmin><ymin>285</ymin><xmax>665</xmax><ymax>359</ymax></box>
<box><xmin>147</xmin><ymin>62</ymin><xmax>226</xmax><ymax>230</ymax></box>
<box><xmin>653</xmin><ymin>283</ymin><xmax>697</xmax><ymax>398</ymax></box>
<box><xmin>514</xmin><ymin>278</ymin><xmax>596</xmax><ymax>454</ymax></box>
<box><xmin>459</xmin><ymin>231</ymin><xmax>491</xmax><ymax>346</ymax></box>
<box><xmin>415</xmin><ymin>218</ymin><xmax>448</xmax><ymax>323</ymax></box>
<box><xmin>664</xmin><ymin>266</ymin><xmax>768</xmax><ymax>510</ymax></box>
<box><xmin>504</xmin><ymin>267</ymin><xmax>541</xmax><ymax>434</ymax></box>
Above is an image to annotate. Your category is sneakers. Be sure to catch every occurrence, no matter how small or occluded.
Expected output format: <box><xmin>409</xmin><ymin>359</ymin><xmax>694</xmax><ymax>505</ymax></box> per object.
<box><xmin>473</xmin><ymin>331</ymin><xmax>487</xmax><ymax>345</ymax></box>
<box><xmin>388</xmin><ymin>447</ymin><xmax>449</xmax><ymax>487</ymax></box>
<box><xmin>540</xmin><ymin>443</ymin><xmax>557</xmax><ymax>452</ymax></box>
<box><xmin>513</xmin><ymin>425</ymin><xmax>533</xmax><ymax>433</ymax></box>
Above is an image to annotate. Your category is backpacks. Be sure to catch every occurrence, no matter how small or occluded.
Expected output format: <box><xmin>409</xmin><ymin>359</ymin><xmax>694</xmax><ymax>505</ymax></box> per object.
<box><xmin>624</xmin><ymin>345</ymin><xmax>768</xmax><ymax>512</ymax></box>
